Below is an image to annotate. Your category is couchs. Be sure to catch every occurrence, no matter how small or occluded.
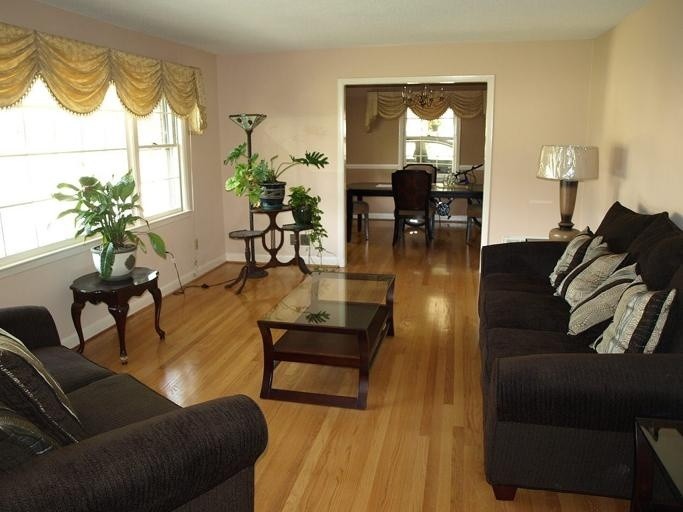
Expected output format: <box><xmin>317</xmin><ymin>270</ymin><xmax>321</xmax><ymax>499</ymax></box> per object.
<box><xmin>476</xmin><ymin>201</ymin><xmax>682</xmax><ymax>504</ymax></box>
<box><xmin>0</xmin><ymin>302</ymin><xmax>269</xmax><ymax>510</ymax></box>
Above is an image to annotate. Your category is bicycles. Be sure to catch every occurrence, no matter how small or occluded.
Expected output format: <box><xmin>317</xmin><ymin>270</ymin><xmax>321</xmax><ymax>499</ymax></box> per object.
<box><xmin>405</xmin><ymin>162</ymin><xmax>483</xmax><ymax>231</ymax></box>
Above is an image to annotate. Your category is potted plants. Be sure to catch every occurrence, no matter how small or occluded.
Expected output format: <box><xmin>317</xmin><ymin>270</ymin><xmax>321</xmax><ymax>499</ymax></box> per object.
<box><xmin>51</xmin><ymin>171</ymin><xmax>170</xmax><ymax>283</ymax></box>
<box><xmin>282</xmin><ymin>183</ymin><xmax>329</xmax><ymax>270</ymax></box>
<box><xmin>223</xmin><ymin>142</ymin><xmax>330</xmax><ymax>209</ymax></box>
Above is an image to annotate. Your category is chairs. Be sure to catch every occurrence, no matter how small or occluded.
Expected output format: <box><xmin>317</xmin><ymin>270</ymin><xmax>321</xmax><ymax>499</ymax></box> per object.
<box><xmin>390</xmin><ymin>168</ymin><xmax>432</xmax><ymax>247</ymax></box>
<box><xmin>399</xmin><ymin>163</ymin><xmax>439</xmax><ymax>240</ymax></box>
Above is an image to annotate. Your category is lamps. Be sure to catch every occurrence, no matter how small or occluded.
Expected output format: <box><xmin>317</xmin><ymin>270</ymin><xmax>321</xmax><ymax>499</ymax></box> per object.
<box><xmin>227</xmin><ymin>111</ymin><xmax>268</xmax><ymax>280</ymax></box>
<box><xmin>534</xmin><ymin>142</ymin><xmax>599</xmax><ymax>239</ymax></box>
<box><xmin>399</xmin><ymin>85</ymin><xmax>448</xmax><ymax>110</ymax></box>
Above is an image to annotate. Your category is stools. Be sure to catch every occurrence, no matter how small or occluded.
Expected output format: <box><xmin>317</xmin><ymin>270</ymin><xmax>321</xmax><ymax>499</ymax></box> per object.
<box><xmin>352</xmin><ymin>199</ymin><xmax>370</xmax><ymax>240</ymax></box>
<box><xmin>464</xmin><ymin>202</ymin><xmax>482</xmax><ymax>246</ymax></box>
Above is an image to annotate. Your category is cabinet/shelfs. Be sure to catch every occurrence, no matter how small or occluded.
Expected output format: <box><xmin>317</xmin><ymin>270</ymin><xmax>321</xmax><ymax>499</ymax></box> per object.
<box><xmin>223</xmin><ymin>204</ymin><xmax>316</xmax><ymax>297</ymax></box>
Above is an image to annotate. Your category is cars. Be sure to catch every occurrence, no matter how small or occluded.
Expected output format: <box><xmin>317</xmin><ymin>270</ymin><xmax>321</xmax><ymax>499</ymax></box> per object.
<box><xmin>406</xmin><ymin>136</ymin><xmax>455</xmax><ymax>173</ymax></box>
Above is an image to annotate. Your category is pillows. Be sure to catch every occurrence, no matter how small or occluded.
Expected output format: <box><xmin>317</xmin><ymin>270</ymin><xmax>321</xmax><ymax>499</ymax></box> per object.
<box><xmin>545</xmin><ymin>225</ymin><xmax>676</xmax><ymax>352</ymax></box>
<box><xmin>0</xmin><ymin>403</ymin><xmax>60</xmax><ymax>459</ymax></box>
<box><xmin>0</xmin><ymin>327</ymin><xmax>83</xmax><ymax>445</ymax></box>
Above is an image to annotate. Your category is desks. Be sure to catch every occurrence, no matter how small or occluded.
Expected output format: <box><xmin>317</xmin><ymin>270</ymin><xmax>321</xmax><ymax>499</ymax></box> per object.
<box><xmin>624</xmin><ymin>415</ymin><xmax>682</xmax><ymax>512</ymax></box>
<box><xmin>69</xmin><ymin>264</ymin><xmax>168</xmax><ymax>367</ymax></box>
<box><xmin>346</xmin><ymin>181</ymin><xmax>483</xmax><ymax>247</ymax></box>
<box><xmin>524</xmin><ymin>237</ymin><xmax>554</xmax><ymax>244</ymax></box>
